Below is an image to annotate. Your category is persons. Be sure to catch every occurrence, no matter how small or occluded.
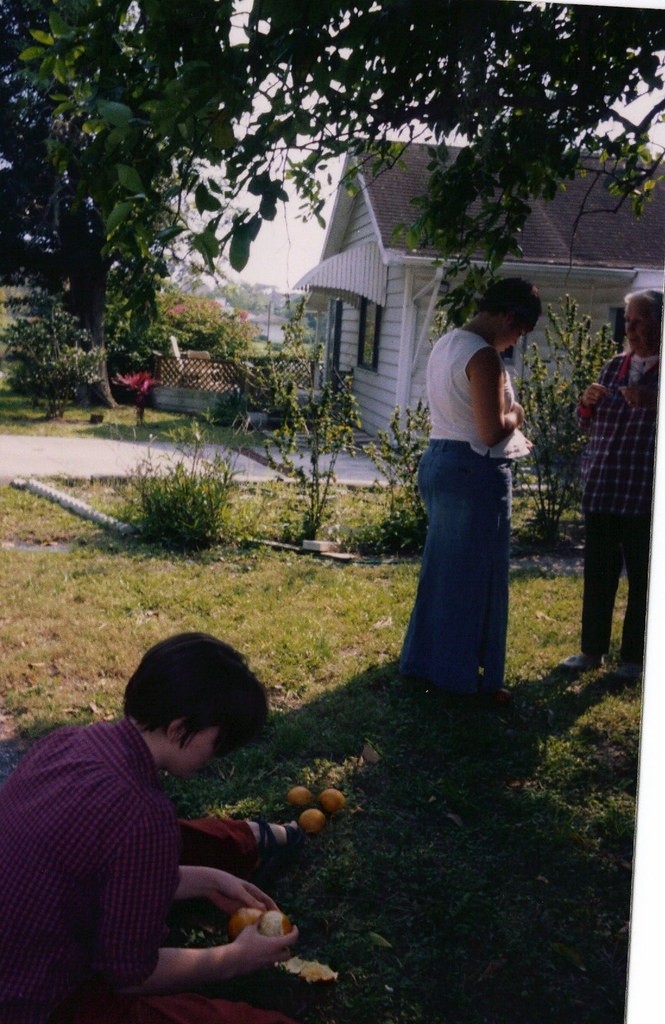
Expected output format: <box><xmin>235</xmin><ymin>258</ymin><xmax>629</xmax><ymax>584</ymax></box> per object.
<box><xmin>400</xmin><ymin>278</ymin><xmax>540</xmax><ymax>705</ymax></box>
<box><xmin>554</xmin><ymin>289</ymin><xmax>664</xmax><ymax>687</ymax></box>
<box><xmin>0</xmin><ymin>632</ymin><xmax>299</xmax><ymax>1024</ymax></box>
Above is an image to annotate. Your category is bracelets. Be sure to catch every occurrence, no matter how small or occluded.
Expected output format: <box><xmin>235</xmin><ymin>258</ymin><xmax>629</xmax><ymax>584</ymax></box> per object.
<box><xmin>579</xmin><ymin>398</ymin><xmax>587</xmax><ymax>409</ymax></box>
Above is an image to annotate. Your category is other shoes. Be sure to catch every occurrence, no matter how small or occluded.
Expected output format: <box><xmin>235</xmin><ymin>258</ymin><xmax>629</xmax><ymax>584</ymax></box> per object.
<box><xmin>558</xmin><ymin>652</ymin><xmax>604</xmax><ymax>672</ymax></box>
<box><xmin>617</xmin><ymin>662</ymin><xmax>642</xmax><ymax>679</ymax></box>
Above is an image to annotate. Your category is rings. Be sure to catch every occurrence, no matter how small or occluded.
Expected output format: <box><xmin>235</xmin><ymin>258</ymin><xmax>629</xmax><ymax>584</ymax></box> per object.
<box><xmin>628</xmin><ymin>400</ymin><xmax>631</xmax><ymax>404</ymax></box>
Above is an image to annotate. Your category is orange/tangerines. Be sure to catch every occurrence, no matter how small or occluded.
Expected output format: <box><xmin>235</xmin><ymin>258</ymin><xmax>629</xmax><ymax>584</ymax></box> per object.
<box><xmin>229</xmin><ymin>907</ymin><xmax>290</xmax><ymax>940</ymax></box>
<box><xmin>298</xmin><ymin>808</ymin><xmax>326</xmax><ymax>833</ymax></box>
<box><xmin>288</xmin><ymin>786</ymin><xmax>313</xmax><ymax>805</ymax></box>
<box><xmin>318</xmin><ymin>788</ymin><xmax>345</xmax><ymax>813</ymax></box>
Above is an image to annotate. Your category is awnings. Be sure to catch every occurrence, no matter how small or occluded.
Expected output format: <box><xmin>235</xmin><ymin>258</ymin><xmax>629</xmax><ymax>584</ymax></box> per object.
<box><xmin>297</xmin><ymin>241</ymin><xmax>387</xmax><ymax>308</ymax></box>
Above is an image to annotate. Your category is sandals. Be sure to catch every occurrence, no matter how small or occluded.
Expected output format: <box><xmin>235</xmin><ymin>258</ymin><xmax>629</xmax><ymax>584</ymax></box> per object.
<box><xmin>242</xmin><ymin>822</ymin><xmax>305</xmax><ymax>885</ymax></box>
